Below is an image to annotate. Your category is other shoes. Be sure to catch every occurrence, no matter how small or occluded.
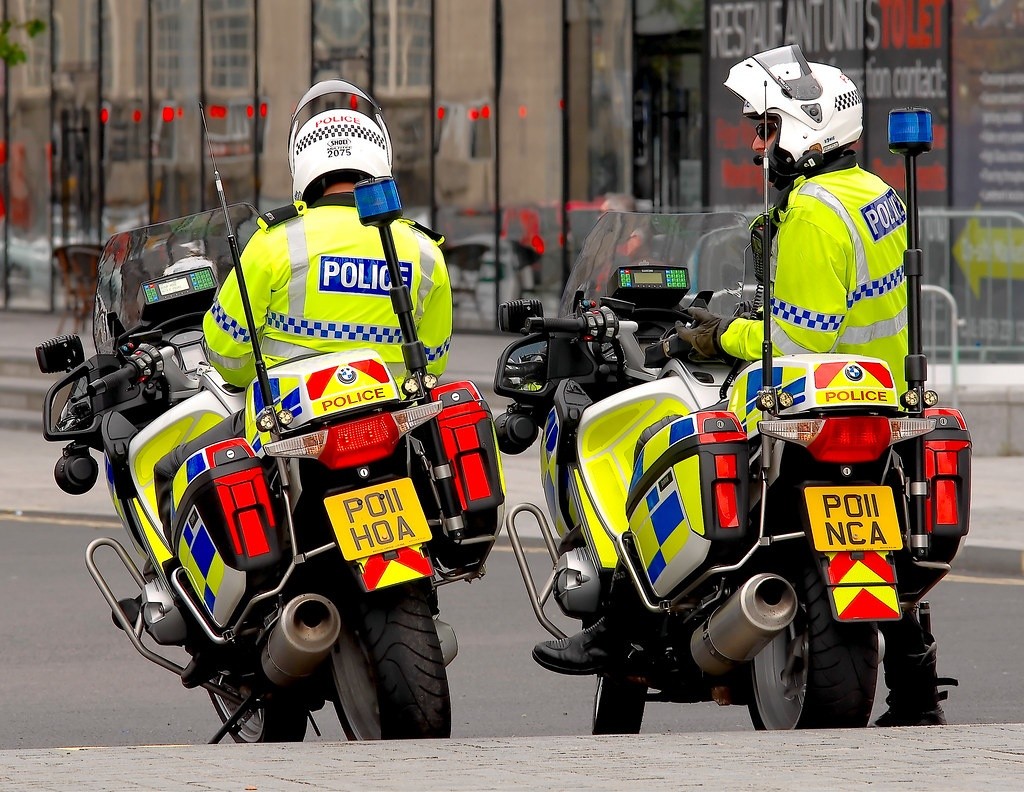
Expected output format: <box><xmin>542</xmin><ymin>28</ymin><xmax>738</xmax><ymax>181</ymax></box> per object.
<box><xmin>179</xmin><ymin>630</ymin><xmax>254</xmax><ymax>689</ymax></box>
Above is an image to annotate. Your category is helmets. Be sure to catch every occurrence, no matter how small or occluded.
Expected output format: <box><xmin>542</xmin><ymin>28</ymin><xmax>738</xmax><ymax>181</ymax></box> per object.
<box><xmin>289</xmin><ymin>109</ymin><xmax>392</xmax><ymax>201</ymax></box>
<box><xmin>744</xmin><ymin>62</ymin><xmax>865</xmax><ymax>169</ymax></box>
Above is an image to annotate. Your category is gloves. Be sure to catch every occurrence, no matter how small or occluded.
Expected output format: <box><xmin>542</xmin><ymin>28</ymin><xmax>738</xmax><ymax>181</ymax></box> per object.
<box><xmin>676</xmin><ymin>305</ymin><xmax>736</xmax><ymax>359</ymax></box>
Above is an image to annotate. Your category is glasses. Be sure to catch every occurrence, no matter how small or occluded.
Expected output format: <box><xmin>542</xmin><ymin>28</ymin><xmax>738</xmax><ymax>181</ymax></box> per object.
<box><xmin>754</xmin><ymin>122</ymin><xmax>781</xmax><ymax>141</ymax></box>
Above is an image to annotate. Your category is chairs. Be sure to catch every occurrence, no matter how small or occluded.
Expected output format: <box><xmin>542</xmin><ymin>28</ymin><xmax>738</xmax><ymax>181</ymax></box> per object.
<box><xmin>442</xmin><ymin>244</ymin><xmax>491</xmax><ymax>330</ymax></box>
<box><xmin>51</xmin><ymin>244</ymin><xmax>104</xmax><ymax>335</ymax></box>
<box><xmin>64</xmin><ymin>246</ymin><xmax>101</xmax><ymax>334</ymax></box>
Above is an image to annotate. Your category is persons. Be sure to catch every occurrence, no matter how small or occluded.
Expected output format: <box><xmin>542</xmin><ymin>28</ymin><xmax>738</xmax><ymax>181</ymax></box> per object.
<box><xmin>151</xmin><ymin>106</ymin><xmax>454</xmax><ymax>560</ymax></box>
<box><xmin>531</xmin><ymin>61</ymin><xmax>951</xmax><ymax>727</ymax></box>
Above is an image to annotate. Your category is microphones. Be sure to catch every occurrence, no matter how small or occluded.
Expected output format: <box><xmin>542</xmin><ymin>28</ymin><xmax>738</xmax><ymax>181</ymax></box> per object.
<box><xmin>753</xmin><ymin>155</ymin><xmax>763</xmax><ymax>166</ymax></box>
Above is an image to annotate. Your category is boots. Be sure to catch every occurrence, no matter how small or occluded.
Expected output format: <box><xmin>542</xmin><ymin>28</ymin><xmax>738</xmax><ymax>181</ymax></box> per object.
<box><xmin>531</xmin><ymin>555</ymin><xmax>635</xmax><ymax>675</ymax></box>
<box><xmin>872</xmin><ymin>635</ymin><xmax>947</xmax><ymax>727</ymax></box>
